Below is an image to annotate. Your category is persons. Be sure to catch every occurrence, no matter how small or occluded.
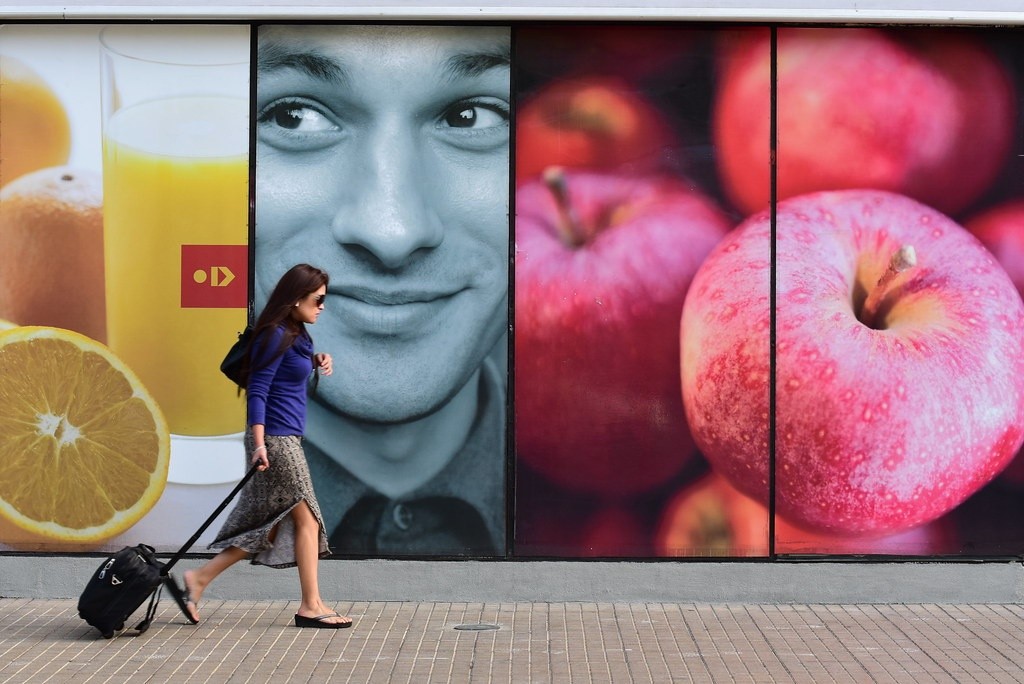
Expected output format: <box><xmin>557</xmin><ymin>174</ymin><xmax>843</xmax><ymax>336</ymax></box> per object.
<box><xmin>255</xmin><ymin>23</ymin><xmax>511</xmax><ymax>556</ymax></box>
<box><xmin>164</xmin><ymin>264</ymin><xmax>352</xmax><ymax>629</ymax></box>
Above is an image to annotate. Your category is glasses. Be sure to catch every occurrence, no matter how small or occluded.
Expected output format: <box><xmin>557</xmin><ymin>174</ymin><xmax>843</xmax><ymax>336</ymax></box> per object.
<box><xmin>305</xmin><ymin>295</ymin><xmax>326</xmax><ymax>308</ymax></box>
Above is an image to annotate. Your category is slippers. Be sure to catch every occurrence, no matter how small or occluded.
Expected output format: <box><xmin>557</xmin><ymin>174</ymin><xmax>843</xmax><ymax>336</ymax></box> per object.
<box><xmin>296</xmin><ymin>611</ymin><xmax>351</xmax><ymax>628</ymax></box>
<box><xmin>164</xmin><ymin>572</ymin><xmax>198</xmax><ymax>624</ymax></box>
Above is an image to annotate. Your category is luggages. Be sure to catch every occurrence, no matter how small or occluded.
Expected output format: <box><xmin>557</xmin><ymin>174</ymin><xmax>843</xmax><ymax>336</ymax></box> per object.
<box><xmin>76</xmin><ymin>457</ymin><xmax>264</xmax><ymax>638</ymax></box>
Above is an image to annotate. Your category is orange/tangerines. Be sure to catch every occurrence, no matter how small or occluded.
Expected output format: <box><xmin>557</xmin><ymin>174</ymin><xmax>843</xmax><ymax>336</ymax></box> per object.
<box><xmin>0</xmin><ymin>325</ymin><xmax>171</xmax><ymax>546</ymax></box>
<box><xmin>0</xmin><ymin>54</ymin><xmax>107</xmax><ymax>345</ymax></box>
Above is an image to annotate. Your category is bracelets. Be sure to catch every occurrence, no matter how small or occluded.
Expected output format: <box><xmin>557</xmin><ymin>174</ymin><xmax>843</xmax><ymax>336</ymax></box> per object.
<box><xmin>252</xmin><ymin>445</ymin><xmax>266</xmax><ymax>455</ymax></box>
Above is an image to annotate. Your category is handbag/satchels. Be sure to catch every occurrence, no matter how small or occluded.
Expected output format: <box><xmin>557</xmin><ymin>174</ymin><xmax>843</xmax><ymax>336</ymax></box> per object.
<box><xmin>220</xmin><ymin>328</ymin><xmax>255</xmax><ymax>391</ymax></box>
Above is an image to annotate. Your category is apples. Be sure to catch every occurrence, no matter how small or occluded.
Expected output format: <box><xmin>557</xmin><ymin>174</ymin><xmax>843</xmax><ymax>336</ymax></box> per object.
<box><xmin>514</xmin><ymin>21</ymin><xmax>1023</xmax><ymax>562</ymax></box>
